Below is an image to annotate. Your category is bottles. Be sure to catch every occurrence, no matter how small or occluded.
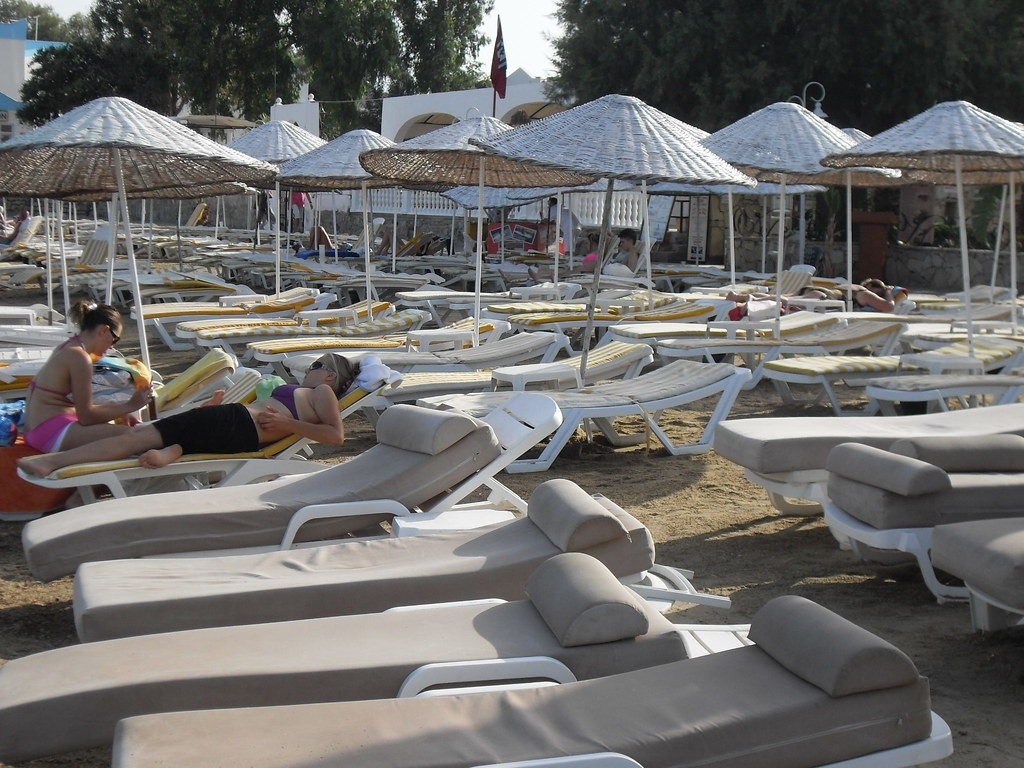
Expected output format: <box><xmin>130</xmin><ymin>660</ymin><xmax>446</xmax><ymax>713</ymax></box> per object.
<box><xmin>28</xmin><ymin>254</ymin><xmax>33</xmax><ymax>264</ymax></box>
<box><xmin>146</xmin><ymin>388</ymin><xmax>158</xmax><ymax>421</ymax></box>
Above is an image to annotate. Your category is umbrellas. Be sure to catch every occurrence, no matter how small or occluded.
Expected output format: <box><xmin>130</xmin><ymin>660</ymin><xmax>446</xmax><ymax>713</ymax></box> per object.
<box><xmin>470</xmin><ymin>93</ymin><xmax>757</xmax><ymax>383</ymax></box>
<box><xmin>701</xmin><ymin>103</ymin><xmax>1024</xmax><ymax>375</ymax></box>
<box><xmin>277</xmin><ymin>127</ymin><xmax>397</xmax><ymax>316</ymax></box>
<box><xmin>0</xmin><ymin>95</ymin><xmax>279</xmax><ymax>368</ymax></box>
<box><xmin>223</xmin><ymin>120</ymin><xmax>329</xmax><ymax>292</ymax></box>
<box><xmin>360</xmin><ymin>116</ymin><xmax>601</xmax><ymax>349</ymax></box>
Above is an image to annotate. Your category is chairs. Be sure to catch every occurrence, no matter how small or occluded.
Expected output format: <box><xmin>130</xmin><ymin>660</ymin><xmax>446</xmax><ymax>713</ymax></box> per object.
<box><xmin>0</xmin><ymin>204</ymin><xmax>1024</xmax><ymax>768</ymax></box>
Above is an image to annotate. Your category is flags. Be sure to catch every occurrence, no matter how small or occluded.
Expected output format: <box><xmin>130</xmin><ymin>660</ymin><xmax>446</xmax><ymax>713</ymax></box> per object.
<box><xmin>490</xmin><ymin>18</ymin><xmax>507</xmax><ymax>100</ymax></box>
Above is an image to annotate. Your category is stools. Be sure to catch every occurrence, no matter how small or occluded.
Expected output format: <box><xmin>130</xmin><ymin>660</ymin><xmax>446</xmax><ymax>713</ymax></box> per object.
<box><xmin>0</xmin><ymin>433</ymin><xmax>72</xmax><ymax>521</ymax></box>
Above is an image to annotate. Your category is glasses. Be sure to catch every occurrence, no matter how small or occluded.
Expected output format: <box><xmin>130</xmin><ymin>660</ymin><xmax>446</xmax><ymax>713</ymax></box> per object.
<box><xmin>309</xmin><ymin>361</ymin><xmax>336</xmax><ymax>373</ymax></box>
<box><xmin>109</xmin><ymin>329</ymin><xmax>120</xmax><ymax>346</ymax></box>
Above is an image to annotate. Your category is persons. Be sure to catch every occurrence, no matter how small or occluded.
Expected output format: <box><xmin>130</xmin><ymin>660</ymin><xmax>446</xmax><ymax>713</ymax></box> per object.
<box><xmin>374</xmin><ymin>230</ymin><xmax>438</xmax><ymax>256</ymax></box>
<box><xmin>618</xmin><ymin>229</ymin><xmax>638</xmax><ymax>273</ymax></box>
<box><xmin>254</xmin><ymin>189</ymin><xmax>275</xmax><ymax>230</ymax></box>
<box><xmin>17</xmin><ymin>351</ymin><xmax>363</xmax><ymax>480</ymax></box>
<box><xmin>527</xmin><ymin>230</ymin><xmax>611</xmax><ymax>283</ymax></box>
<box><xmin>0</xmin><ymin>206</ymin><xmax>13</xmax><ymax>233</ymax></box>
<box><xmin>287</xmin><ymin>190</ymin><xmax>313</xmax><ymax>234</ymax></box>
<box><xmin>306</xmin><ymin>226</ymin><xmax>353</xmax><ymax>251</ymax></box>
<box><xmin>547</xmin><ymin>197</ymin><xmax>581</xmax><ymax>252</ymax></box>
<box><xmin>727</xmin><ymin>278</ymin><xmax>895</xmax><ymax>313</ymax></box>
<box><xmin>0</xmin><ymin>211</ymin><xmax>29</xmax><ymax>245</ymax></box>
<box><xmin>24</xmin><ymin>301</ymin><xmax>157</xmax><ymax>509</ymax></box>
<box><xmin>194</xmin><ymin>198</ymin><xmax>210</xmax><ymax>226</ymax></box>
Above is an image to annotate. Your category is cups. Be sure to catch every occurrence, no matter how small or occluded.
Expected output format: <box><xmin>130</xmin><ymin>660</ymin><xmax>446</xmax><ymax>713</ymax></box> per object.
<box><xmin>36</xmin><ymin>261</ymin><xmax>41</xmax><ymax>267</ymax></box>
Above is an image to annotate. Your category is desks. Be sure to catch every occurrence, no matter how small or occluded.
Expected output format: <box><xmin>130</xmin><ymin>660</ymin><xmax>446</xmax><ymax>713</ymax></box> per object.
<box><xmin>406</xmin><ymin>330</ymin><xmax>478</xmax><ymax>352</ymax></box>
<box><xmin>219</xmin><ymin>293</ymin><xmax>268</xmax><ymax>307</ymax></box>
<box><xmin>296</xmin><ymin>308</ymin><xmax>360</xmax><ymax>328</ymax></box>
<box><xmin>490</xmin><ymin>364</ymin><xmax>591</xmax><ymax>442</ymax></box>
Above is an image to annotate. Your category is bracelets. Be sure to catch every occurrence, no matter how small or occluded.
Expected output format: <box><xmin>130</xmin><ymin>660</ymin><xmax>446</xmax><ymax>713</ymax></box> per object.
<box><xmin>310</xmin><ymin>202</ymin><xmax>312</xmax><ymax>203</ymax></box>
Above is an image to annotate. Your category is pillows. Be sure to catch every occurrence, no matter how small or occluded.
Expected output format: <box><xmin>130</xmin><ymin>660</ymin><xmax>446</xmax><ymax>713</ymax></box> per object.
<box><xmin>892</xmin><ymin>434</ymin><xmax>1024</xmax><ymax>472</ymax></box>
<box><xmin>746</xmin><ymin>594</ymin><xmax>920</xmax><ymax>700</ymax></box>
<box><xmin>524</xmin><ymin>552</ymin><xmax>651</xmax><ymax>647</ymax></box>
<box><xmin>527</xmin><ymin>478</ymin><xmax>625</xmax><ymax>553</ymax></box>
<box><xmin>376</xmin><ymin>405</ymin><xmax>476</xmax><ymax>455</ymax></box>
<box><xmin>826</xmin><ymin>444</ymin><xmax>954</xmax><ymax>494</ymax></box>
